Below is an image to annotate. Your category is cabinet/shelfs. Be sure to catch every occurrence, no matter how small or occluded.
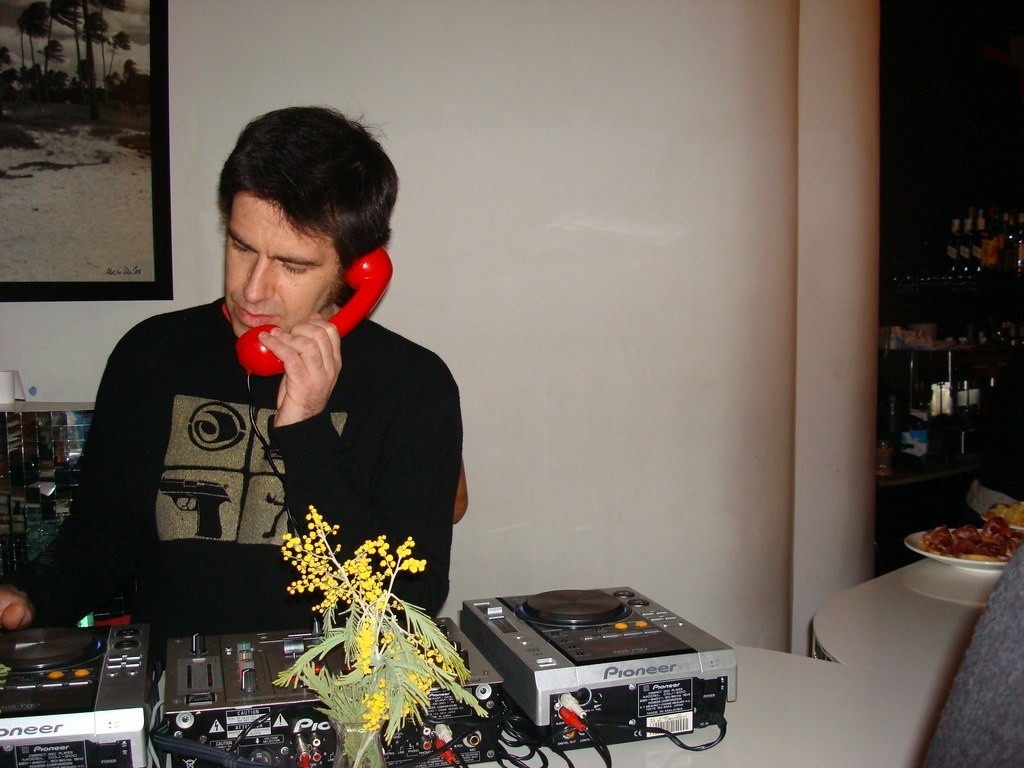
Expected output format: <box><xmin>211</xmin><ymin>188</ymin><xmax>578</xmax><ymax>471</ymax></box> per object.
<box><xmin>875</xmin><ymin>208</ymin><xmax>1024</xmax><ymax>568</ymax></box>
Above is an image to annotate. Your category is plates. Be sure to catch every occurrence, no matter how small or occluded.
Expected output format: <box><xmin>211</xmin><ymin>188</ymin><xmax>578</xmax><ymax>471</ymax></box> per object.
<box><xmin>904</xmin><ymin>528</ymin><xmax>1024</xmax><ymax>572</ymax></box>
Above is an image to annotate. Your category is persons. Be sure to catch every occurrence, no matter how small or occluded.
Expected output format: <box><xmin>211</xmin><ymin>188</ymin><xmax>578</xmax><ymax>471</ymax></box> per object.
<box><xmin>973</xmin><ymin>343</ymin><xmax>1024</xmax><ymax>502</ymax></box>
<box><xmin>0</xmin><ymin>106</ymin><xmax>462</xmax><ymax>633</ymax></box>
<box><xmin>922</xmin><ymin>541</ymin><xmax>1024</xmax><ymax>768</ymax></box>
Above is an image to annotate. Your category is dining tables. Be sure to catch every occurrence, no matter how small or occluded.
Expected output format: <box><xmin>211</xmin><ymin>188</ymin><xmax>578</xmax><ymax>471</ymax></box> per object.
<box><xmin>811</xmin><ymin>502</ymin><xmax>1024</xmax><ymax>694</ymax></box>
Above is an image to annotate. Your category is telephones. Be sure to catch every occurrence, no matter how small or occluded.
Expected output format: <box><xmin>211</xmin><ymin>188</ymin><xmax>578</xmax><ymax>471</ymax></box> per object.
<box><xmin>235</xmin><ymin>245</ymin><xmax>394</xmax><ymax>378</ymax></box>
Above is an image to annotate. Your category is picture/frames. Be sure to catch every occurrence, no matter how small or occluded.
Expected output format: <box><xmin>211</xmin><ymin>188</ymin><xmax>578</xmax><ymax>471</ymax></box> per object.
<box><xmin>0</xmin><ymin>0</ymin><xmax>174</xmax><ymax>302</ymax></box>
